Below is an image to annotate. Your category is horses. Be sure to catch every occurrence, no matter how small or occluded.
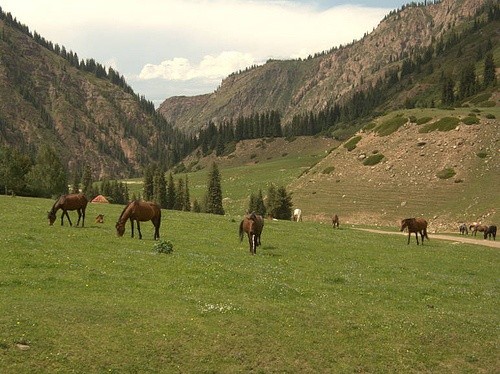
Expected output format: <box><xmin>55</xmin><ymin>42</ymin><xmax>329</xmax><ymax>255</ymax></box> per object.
<box><xmin>238</xmin><ymin>208</ymin><xmax>264</xmax><ymax>256</ymax></box>
<box><xmin>400</xmin><ymin>217</ymin><xmax>430</xmax><ymax>246</ymax></box>
<box><xmin>115</xmin><ymin>199</ymin><xmax>162</xmax><ymax>241</ymax></box>
<box><xmin>46</xmin><ymin>193</ymin><xmax>89</xmax><ymax>228</ymax></box>
<box><xmin>459</xmin><ymin>223</ymin><xmax>497</xmax><ymax>241</ymax></box>
